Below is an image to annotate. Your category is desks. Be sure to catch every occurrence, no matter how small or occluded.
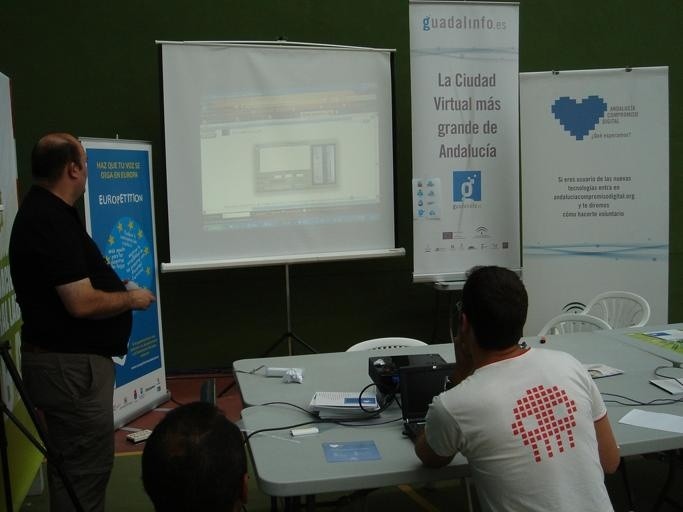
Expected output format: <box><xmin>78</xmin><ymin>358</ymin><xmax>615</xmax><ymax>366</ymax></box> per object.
<box><xmin>233</xmin><ymin>323</ymin><xmax>682</xmax><ymax>512</ymax></box>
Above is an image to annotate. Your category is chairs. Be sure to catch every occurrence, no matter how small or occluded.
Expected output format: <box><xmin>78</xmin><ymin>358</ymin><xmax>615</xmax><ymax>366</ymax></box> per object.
<box><xmin>536</xmin><ymin>291</ymin><xmax>651</xmax><ymax>338</ymax></box>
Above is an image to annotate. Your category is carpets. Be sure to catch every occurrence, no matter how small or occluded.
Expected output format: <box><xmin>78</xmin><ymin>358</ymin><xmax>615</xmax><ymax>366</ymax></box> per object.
<box><xmin>117</xmin><ymin>374</ymin><xmax>249</xmax><ymax>453</ymax></box>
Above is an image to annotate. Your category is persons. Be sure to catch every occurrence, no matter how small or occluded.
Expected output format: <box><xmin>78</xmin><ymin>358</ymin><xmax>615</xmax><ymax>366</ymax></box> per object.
<box><xmin>142</xmin><ymin>402</ymin><xmax>250</xmax><ymax>512</ymax></box>
<box><xmin>414</xmin><ymin>265</ymin><xmax>621</xmax><ymax>512</ymax></box>
<box><xmin>9</xmin><ymin>133</ymin><xmax>156</xmax><ymax>512</ymax></box>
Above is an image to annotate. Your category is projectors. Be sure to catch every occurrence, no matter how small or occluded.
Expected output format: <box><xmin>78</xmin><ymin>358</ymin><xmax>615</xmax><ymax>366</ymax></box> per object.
<box><xmin>368</xmin><ymin>352</ymin><xmax>447</xmax><ymax>395</ymax></box>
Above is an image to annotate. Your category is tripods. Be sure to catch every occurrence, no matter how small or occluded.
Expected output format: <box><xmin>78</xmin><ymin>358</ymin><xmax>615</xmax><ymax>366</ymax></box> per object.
<box><xmin>216</xmin><ymin>263</ymin><xmax>318</xmax><ymax>399</ymax></box>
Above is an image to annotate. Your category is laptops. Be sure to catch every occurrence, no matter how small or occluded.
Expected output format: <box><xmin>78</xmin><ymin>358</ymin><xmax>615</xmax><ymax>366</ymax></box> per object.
<box><xmin>399</xmin><ymin>362</ymin><xmax>465</xmax><ymax>445</ymax></box>
